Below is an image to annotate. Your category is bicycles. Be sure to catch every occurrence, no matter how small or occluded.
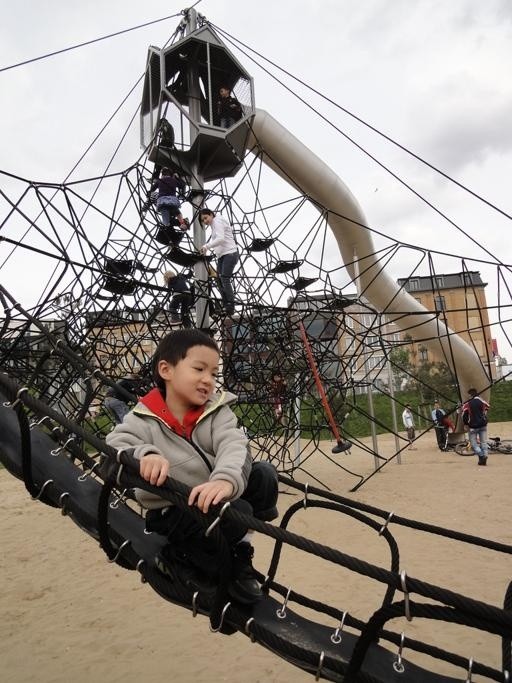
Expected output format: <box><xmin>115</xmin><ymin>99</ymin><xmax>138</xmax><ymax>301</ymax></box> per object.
<box><xmin>455</xmin><ymin>437</ymin><xmax>512</xmax><ymax>456</ymax></box>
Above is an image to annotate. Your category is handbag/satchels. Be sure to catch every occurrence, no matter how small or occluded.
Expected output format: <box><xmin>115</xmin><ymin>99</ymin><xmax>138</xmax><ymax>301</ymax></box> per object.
<box><xmin>478</xmin><ymin>414</ymin><xmax>488</xmax><ymax>425</ymax></box>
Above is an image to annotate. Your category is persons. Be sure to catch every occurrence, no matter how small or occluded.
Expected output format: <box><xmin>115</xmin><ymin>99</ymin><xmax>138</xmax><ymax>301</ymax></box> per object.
<box><xmin>463</xmin><ymin>388</ymin><xmax>490</xmax><ymax>465</ymax></box>
<box><xmin>199</xmin><ymin>207</ymin><xmax>239</xmax><ymax>317</ymax></box>
<box><xmin>163</xmin><ymin>270</ymin><xmax>193</xmax><ymax>329</ymax></box>
<box><xmin>150</xmin><ymin>166</ymin><xmax>189</xmax><ymax>230</ymax></box>
<box><xmin>157</xmin><ymin>116</ymin><xmax>174</xmax><ymax>149</ymax></box>
<box><xmin>402</xmin><ymin>404</ymin><xmax>418</xmax><ymax>450</ymax></box>
<box><xmin>432</xmin><ymin>400</ymin><xmax>449</xmax><ymax>450</ymax></box>
<box><xmin>107</xmin><ymin>327</ymin><xmax>280</xmax><ymax>604</ymax></box>
<box><xmin>106</xmin><ymin>373</ymin><xmax>140</xmax><ymax>425</ymax></box>
<box><xmin>219</xmin><ymin>82</ymin><xmax>242</xmax><ymax>128</ymax></box>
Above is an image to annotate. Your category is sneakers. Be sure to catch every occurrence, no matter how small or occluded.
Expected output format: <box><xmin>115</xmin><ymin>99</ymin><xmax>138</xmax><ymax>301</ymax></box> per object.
<box><xmin>214</xmin><ymin>308</ymin><xmax>234</xmax><ymax>316</ymax></box>
<box><xmin>180</xmin><ymin>224</ymin><xmax>188</xmax><ymax>230</ymax></box>
<box><xmin>408</xmin><ymin>446</ymin><xmax>418</xmax><ymax>450</ymax></box>
<box><xmin>162</xmin><ymin>543</ymin><xmax>219</xmax><ymax>595</ymax></box>
<box><xmin>478</xmin><ymin>456</ymin><xmax>488</xmax><ymax>465</ymax></box>
<box><xmin>225</xmin><ymin>547</ymin><xmax>259</xmax><ymax>603</ymax></box>
